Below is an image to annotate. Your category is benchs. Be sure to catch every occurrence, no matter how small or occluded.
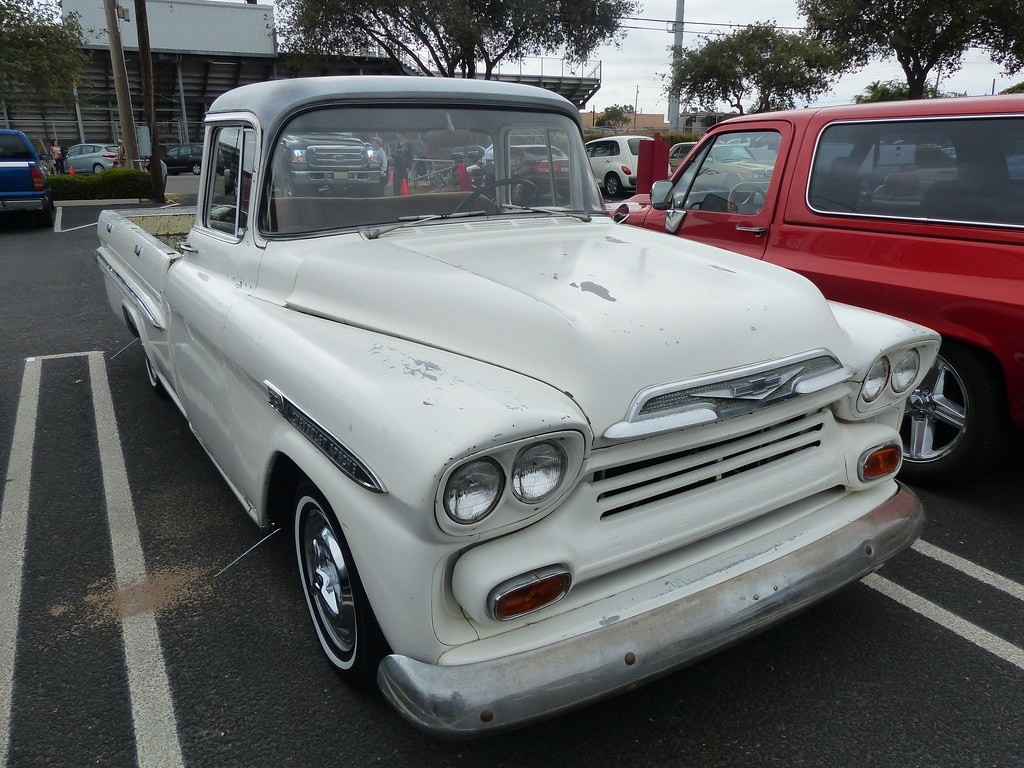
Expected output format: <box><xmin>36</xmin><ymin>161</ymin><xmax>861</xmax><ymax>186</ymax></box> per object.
<box><xmin>235</xmin><ymin>190</ymin><xmax>500</xmax><ymax>232</ymax></box>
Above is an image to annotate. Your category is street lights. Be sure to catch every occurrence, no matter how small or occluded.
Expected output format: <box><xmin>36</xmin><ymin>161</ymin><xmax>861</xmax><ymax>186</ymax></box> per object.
<box><xmin>715</xmin><ymin>112</ymin><xmax>725</xmax><ymax>124</ymax></box>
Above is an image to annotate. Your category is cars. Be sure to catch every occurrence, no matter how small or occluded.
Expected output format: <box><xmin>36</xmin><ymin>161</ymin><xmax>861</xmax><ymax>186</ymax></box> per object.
<box><xmin>62</xmin><ymin>143</ymin><xmax>119</xmax><ymax>174</ymax></box>
<box><xmin>604</xmin><ymin>93</ymin><xmax>1024</xmax><ymax>488</ymax></box>
<box><xmin>726</xmin><ymin>137</ymin><xmax>752</xmax><ymax>147</ymax></box>
<box><xmin>859</xmin><ymin>147</ymin><xmax>958</xmax><ymax>209</ymax></box>
<box><xmin>162</xmin><ymin>143</ymin><xmax>229</xmax><ymax>175</ymax></box>
<box><xmin>668</xmin><ymin>142</ymin><xmax>775</xmax><ymax>192</ymax></box>
<box><xmin>451</xmin><ymin>144</ymin><xmax>487</xmax><ymax>163</ymax></box>
<box><xmin>216</xmin><ymin>125</ymin><xmax>381</xmax><ymax>198</ymax></box>
<box><xmin>585</xmin><ymin>135</ymin><xmax>672</xmax><ymax>199</ymax></box>
<box><xmin>0</xmin><ymin>130</ymin><xmax>56</xmax><ymax>227</ymax></box>
<box><xmin>465</xmin><ymin>145</ymin><xmax>570</xmax><ymax>200</ymax></box>
<box><xmin>387</xmin><ymin>139</ymin><xmax>427</xmax><ymax>159</ymax></box>
<box><xmin>93</xmin><ymin>75</ymin><xmax>941</xmax><ymax>738</ymax></box>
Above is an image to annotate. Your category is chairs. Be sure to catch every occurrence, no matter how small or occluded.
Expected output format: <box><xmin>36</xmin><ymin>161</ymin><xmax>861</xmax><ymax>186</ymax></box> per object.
<box><xmin>830</xmin><ymin>155</ymin><xmax>861</xmax><ymax>209</ymax></box>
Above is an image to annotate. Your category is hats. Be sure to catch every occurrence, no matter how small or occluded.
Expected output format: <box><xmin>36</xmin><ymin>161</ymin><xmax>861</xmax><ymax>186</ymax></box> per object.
<box><xmin>117</xmin><ymin>140</ymin><xmax>123</xmax><ymax>143</ymax></box>
<box><xmin>372</xmin><ymin>137</ymin><xmax>384</xmax><ymax>146</ymax></box>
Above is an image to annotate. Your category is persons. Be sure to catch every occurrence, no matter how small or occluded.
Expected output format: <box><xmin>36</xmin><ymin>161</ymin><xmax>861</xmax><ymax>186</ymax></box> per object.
<box><xmin>160</xmin><ymin>148</ymin><xmax>167</xmax><ymax>196</ymax></box>
<box><xmin>390</xmin><ymin>129</ymin><xmax>413</xmax><ymax>196</ymax></box>
<box><xmin>369</xmin><ymin>136</ymin><xmax>388</xmax><ymax>189</ymax></box>
<box><xmin>476</xmin><ymin>135</ymin><xmax>496</xmax><ymax>198</ymax></box>
<box><xmin>116</xmin><ymin>140</ymin><xmax>126</xmax><ymax>170</ymax></box>
<box><xmin>50</xmin><ymin>139</ymin><xmax>66</xmax><ymax>175</ymax></box>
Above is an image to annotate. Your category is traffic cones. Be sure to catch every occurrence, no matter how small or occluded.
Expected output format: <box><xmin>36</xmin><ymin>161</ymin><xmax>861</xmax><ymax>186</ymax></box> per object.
<box><xmin>401</xmin><ymin>179</ymin><xmax>409</xmax><ymax>196</ymax></box>
<box><xmin>70</xmin><ymin>166</ymin><xmax>74</xmax><ymax>175</ymax></box>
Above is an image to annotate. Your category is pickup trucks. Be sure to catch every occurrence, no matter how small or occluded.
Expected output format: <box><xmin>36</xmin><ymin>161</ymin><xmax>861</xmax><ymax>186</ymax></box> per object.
<box><xmin>747</xmin><ymin>131</ymin><xmax>916</xmax><ymax>178</ymax></box>
